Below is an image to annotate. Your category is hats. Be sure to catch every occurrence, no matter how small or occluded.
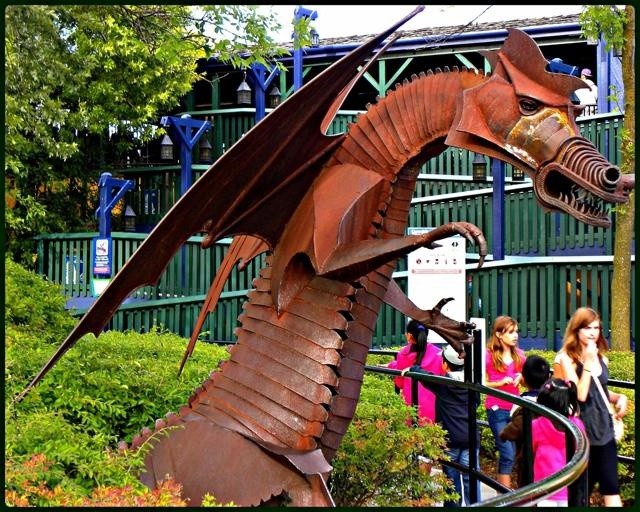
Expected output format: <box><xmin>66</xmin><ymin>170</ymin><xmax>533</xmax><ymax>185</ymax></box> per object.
<box><xmin>437</xmin><ymin>344</ymin><xmax>465</xmax><ymax>367</ymax></box>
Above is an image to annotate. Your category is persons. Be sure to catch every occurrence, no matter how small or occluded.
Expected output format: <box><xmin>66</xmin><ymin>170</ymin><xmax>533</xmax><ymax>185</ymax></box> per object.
<box><xmin>574</xmin><ymin>67</ymin><xmax>598</xmax><ymax>116</ymax></box>
<box><xmin>484</xmin><ymin>307</ymin><xmax>629</xmax><ymax>507</ymax></box>
<box><xmin>377</xmin><ymin>318</ymin><xmax>483</xmax><ymax>507</ymax></box>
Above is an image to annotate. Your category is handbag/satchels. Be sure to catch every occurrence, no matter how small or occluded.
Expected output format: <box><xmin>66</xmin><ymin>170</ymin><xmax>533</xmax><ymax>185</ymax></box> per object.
<box><xmin>610</xmin><ymin>411</ymin><xmax>624</xmax><ymax>444</ymax></box>
